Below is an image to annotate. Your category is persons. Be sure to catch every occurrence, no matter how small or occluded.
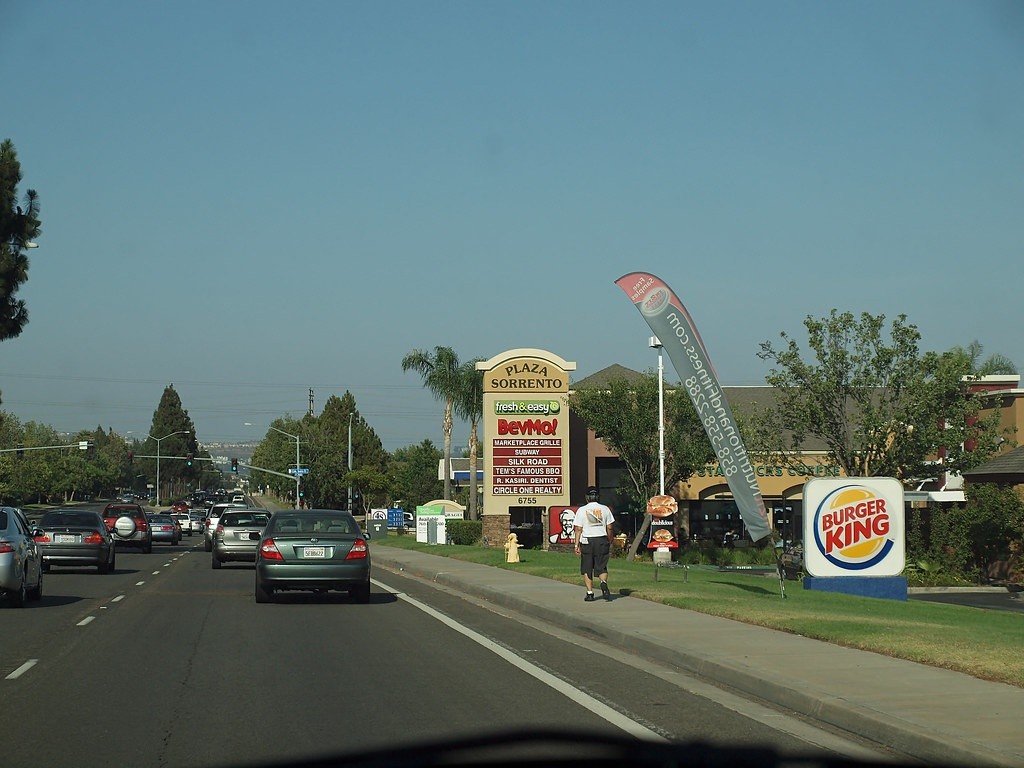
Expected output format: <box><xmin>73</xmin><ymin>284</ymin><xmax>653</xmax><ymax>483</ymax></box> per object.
<box><xmin>618</xmin><ymin>529</ymin><xmax>740</xmax><ymax>545</ymax></box>
<box><xmin>573</xmin><ymin>486</ymin><xmax>617</xmax><ymax>602</ymax></box>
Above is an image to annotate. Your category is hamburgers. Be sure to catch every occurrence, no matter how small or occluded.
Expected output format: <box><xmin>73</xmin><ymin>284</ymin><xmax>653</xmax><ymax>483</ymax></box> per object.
<box><xmin>647</xmin><ymin>495</ymin><xmax>679</xmax><ymax>517</ymax></box>
<box><xmin>652</xmin><ymin>529</ymin><xmax>674</xmax><ymax>543</ymax></box>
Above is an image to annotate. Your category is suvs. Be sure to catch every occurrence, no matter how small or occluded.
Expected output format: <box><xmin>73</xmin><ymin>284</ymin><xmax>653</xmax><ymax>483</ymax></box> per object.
<box><xmin>102</xmin><ymin>503</ymin><xmax>153</xmax><ymax>554</ymax></box>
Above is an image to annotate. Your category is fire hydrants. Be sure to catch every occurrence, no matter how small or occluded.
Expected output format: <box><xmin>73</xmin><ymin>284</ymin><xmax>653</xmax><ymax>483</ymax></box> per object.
<box><xmin>504</xmin><ymin>532</ymin><xmax>524</xmax><ymax>563</ymax></box>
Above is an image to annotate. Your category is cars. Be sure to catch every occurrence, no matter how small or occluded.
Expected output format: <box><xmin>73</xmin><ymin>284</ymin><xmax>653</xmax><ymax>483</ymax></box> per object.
<box><xmin>145</xmin><ymin>514</ymin><xmax>180</xmax><ymax>546</ymax></box>
<box><xmin>168</xmin><ymin>487</ymin><xmax>252</xmax><ymax>553</ymax></box>
<box><xmin>402</xmin><ymin>512</ymin><xmax>413</xmax><ymax>529</ymax></box>
<box><xmin>0</xmin><ymin>505</ymin><xmax>46</xmax><ymax>607</ymax></box>
<box><xmin>210</xmin><ymin>507</ymin><xmax>273</xmax><ymax>570</ymax></box>
<box><xmin>31</xmin><ymin>509</ymin><xmax>118</xmax><ymax>574</ymax></box>
<box><xmin>248</xmin><ymin>509</ymin><xmax>372</xmax><ymax>603</ymax></box>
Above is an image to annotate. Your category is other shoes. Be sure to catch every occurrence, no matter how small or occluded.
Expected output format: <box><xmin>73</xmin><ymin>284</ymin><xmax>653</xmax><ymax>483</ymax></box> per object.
<box><xmin>600</xmin><ymin>580</ymin><xmax>610</xmax><ymax>599</ymax></box>
<box><xmin>584</xmin><ymin>591</ymin><xmax>595</xmax><ymax>601</ymax></box>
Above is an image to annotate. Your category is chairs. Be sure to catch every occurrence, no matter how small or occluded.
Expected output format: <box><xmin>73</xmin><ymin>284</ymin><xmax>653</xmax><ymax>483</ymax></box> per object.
<box><xmin>328</xmin><ymin>526</ymin><xmax>345</xmax><ymax>532</ymax></box>
<box><xmin>281</xmin><ymin>526</ymin><xmax>298</xmax><ymax>532</ymax></box>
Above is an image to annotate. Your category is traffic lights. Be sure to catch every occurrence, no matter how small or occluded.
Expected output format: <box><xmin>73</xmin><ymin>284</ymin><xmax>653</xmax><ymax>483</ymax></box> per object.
<box><xmin>299</xmin><ymin>485</ymin><xmax>304</xmax><ymax>496</ymax></box>
<box><xmin>231</xmin><ymin>456</ymin><xmax>237</xmax><ymax>472</ymax></box>
<box><xmin>127</xmin><ymin>451</ymin><xmax>132</xmax><ymax>463</ymax></box>
<box><xmin>187</xmin><ymin>453</ymin><xmax>192</xmax><ymax>467</ymax></box>
<box><xmin>299</xmin><ymin>501</ymin><xmax>303</xmax><ymax>506</ymax></box>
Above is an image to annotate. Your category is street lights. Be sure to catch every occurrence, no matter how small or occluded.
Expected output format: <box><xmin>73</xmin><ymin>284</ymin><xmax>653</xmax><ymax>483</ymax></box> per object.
<box><xmin>127</xmin><ymin>430</ymin><xmax>191</xmax><ymax>506</ymax></box>
<box><xmin>244</xmin><ymin>422</ymin><xmax>301</xmax><ymax>509</ymax></box>
<box><xmin>348</xmin><ymin>413</ymin><xmax>354</xmax><ymax>510</ymax></box>
<box><xmin>648</xmin><ymin>335</ymin><xmax>665</xmax><ymax>496</ymax></box>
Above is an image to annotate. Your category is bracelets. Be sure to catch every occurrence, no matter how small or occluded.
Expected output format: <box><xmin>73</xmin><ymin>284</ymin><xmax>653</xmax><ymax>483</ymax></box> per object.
<box><xmin>574</xmin><ymin>544</ymin><xmax>579</xmax><ymax>547</ymax></box>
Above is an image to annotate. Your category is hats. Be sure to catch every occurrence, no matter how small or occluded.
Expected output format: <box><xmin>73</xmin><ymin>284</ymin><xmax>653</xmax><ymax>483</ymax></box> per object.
<box><xmin>585</xmin><ymin>486</ymin><xmax>599</xmax><ymax>496</ymax></box>
